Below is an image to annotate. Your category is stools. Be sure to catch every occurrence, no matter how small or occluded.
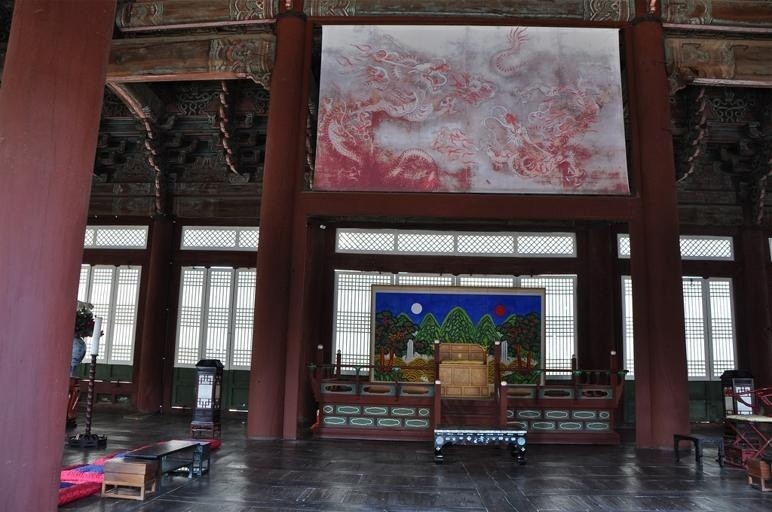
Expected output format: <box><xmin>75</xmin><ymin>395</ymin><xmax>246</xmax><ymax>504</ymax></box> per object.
<box><xmin>670</xmin><ymin>431</ymin><xmax>726</xmax><ymax>469</ymax></box>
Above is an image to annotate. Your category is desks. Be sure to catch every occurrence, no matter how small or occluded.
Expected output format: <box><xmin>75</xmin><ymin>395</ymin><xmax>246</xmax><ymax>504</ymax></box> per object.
<box><xmin>98</xmin><ymin>438</ymin><xmax>210</xmax><ymax>501</ymax></box>
<box><xmin>432</xmin><ymin>423</ymin><xmax>528</xmax><ymax>465</ymax></box>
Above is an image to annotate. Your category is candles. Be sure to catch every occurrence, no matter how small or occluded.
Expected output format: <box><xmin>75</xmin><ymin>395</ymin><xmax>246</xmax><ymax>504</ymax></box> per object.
<box><xmin>90</xmin><ymin>316</ymin><xmax>103</xmax><ymax>357</ymax></box>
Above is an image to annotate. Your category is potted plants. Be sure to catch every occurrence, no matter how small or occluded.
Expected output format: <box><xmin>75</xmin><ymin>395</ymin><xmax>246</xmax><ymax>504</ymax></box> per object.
<box><xmin>69</xmin><ymin>304</ymin><xmax>94</xmax><ymax>380</ymax></box>
